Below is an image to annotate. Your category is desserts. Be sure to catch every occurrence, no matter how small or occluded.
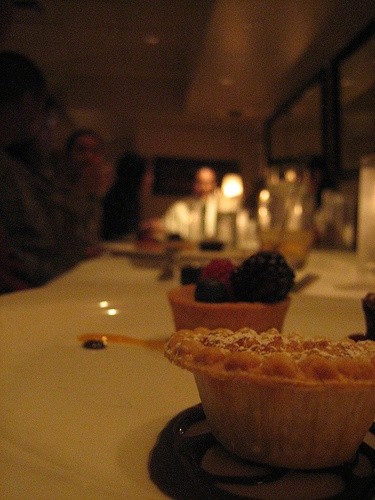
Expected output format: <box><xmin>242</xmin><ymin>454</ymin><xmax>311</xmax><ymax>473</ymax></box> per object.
<box><xmin>163</xmin><ymin>326</ymin><xmax>375</xmax><ymax>471</ymax></box>
<box><xmin>164</xmin><ymin>233</ymin><xmax>295</xmax><ymax>333</ymax></box>
<box><xmin>361</xmin><ymin>291</ymin><xmax>375</xmax><ymax>341</ymax></box>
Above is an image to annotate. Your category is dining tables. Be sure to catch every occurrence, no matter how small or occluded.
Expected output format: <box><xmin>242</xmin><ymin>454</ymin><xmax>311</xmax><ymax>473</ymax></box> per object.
<box><xmin>0</xmin><ymin>233</ymin><xmax>375</xmax><ymax>500</ymax></box>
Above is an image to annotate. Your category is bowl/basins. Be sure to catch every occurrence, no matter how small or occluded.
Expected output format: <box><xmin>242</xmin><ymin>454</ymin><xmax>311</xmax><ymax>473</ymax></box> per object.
<box><xmin>166</xmin><ymin>284</ymin><xmax>291</xmax><ymax>337</ymax></box>
<box><xmin>184</xmin><ymin>364</ymin><xmax>375</xmax><ymax>469</ymax></box>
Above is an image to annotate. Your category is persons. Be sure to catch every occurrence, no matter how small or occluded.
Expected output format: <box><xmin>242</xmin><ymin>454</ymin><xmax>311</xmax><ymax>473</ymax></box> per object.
<box><xmin>99</xmin><ymin>152</ymin><xmax>152</xmax><ymax>243</ymax></box>
<box><xmin>140</xmin><ymin>166</ymin><xmax>250</xmax><ymax>244</ymax></box>
<box><xmin>0</xmin><ymin>48</ymin><xmax>103</xmax><ymax>296</ymax></box>
<box><xmin>68</xmin><ymin>127</ymin><xmax>112</xmax><ymax>237</ymax></box>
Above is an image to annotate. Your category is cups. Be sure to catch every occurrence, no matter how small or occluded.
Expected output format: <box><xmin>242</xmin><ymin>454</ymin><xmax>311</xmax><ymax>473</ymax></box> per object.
<box><xmin>320</xmin><ymin>190</ymin><xmax>355</xmax><ymax>252</ymax></box>
<box><xmin>186</xmin><ymin>199</ymin><xmax>206</xmax><ymax>240</ymax></box>
<box><xmin>213</xmin><ymin>211</ymin><xmax>238</xmax><ymax>247</ymax></box>
<box><xmin>268</xmin><ymin>181</ymin><xmax>313</xmax><ymax>268</ymax></box>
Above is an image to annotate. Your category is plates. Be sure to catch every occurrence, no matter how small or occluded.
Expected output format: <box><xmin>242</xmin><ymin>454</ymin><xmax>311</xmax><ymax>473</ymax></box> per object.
<box><xmin>108</xmin><ymin>241</ymin><xmax>164</xmax><ymax>258</ymax></box>
